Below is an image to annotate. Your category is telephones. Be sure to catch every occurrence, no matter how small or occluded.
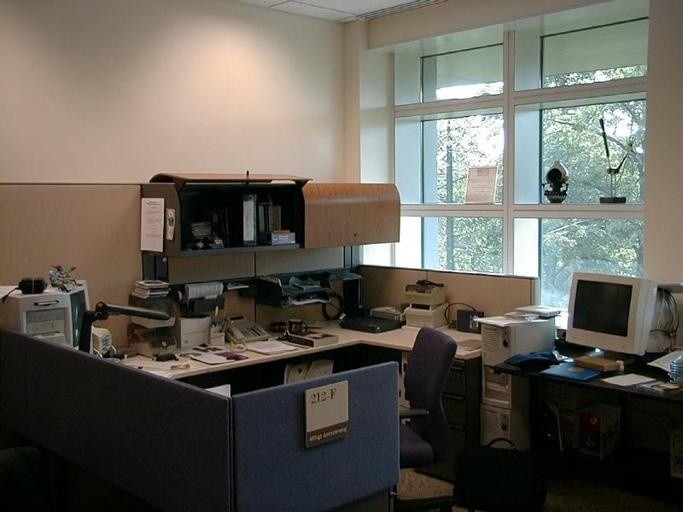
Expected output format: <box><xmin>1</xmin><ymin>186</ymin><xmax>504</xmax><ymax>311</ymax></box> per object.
<box><xmin>224</xmin><ymin>314</ymin><xmax>271</xmax><ymax>352</ymax></box>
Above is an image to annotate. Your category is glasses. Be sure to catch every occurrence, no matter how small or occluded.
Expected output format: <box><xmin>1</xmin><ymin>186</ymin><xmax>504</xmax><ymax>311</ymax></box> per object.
<box><xmin>291</xmin><ymin>323</ymin><xmax>322</xmax><ymax>334</ymax></box>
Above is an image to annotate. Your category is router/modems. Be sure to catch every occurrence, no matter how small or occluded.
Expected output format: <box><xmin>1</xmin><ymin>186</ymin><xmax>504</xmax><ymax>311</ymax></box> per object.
<box><xmin>510</xmin><ymin>304</ymin><xmax>561</xmax><ymax>319</ymax></box>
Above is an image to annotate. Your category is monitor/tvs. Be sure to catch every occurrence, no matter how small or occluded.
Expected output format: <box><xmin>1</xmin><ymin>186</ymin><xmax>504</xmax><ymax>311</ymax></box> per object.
<box><xmin>52</xmin><ymin>279</ymin><xmax>91</xmax><ymax>351</ymax></box>
<box><xmin>565</xmin><ymin>271</ymin><xmax>658</xmax><ymax>356</ymax></box>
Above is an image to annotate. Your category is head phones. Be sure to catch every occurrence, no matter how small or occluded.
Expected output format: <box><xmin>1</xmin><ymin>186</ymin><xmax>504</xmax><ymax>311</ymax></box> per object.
<box><xmin>18</xmin><ymin>278</ymin><xmax>46</xmax><ymax>294</ymax></box>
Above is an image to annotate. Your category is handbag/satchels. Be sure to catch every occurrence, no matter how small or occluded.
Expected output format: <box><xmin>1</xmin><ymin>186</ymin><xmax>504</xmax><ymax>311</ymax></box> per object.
<box><xmin>452</xmin><ymin>437</ymin><xmax>549</xmax><ymax>512</ymax></box>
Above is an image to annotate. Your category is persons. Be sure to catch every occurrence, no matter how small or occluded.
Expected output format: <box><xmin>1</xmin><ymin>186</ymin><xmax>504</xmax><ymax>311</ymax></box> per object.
<box><xmin>48</xmin><ymin>264</ymin><xmax>85</xmax><ymax>294</ymax></box>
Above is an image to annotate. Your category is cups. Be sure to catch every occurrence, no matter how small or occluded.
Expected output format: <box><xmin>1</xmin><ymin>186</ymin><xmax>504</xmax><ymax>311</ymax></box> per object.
<box><xmin>288</xmin><ymin>318</ymin><xmax>308</xmax><ymax>336</ymax></box>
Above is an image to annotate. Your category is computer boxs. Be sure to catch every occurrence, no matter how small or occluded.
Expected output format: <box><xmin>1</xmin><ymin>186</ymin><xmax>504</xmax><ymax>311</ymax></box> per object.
<box><xmin>0</xmin><ymin>286</ymin><xmax>73</xmax><ymax>348</ymax></box>
<box><xmin>480</xmin><ymin>406</ymin><xmax>528</xmax><ymax>450</ymax></box>
<box><xmin>481</xmin><ymin>316</ymin><xmax>555</xmax><ymax>408</ymax></box>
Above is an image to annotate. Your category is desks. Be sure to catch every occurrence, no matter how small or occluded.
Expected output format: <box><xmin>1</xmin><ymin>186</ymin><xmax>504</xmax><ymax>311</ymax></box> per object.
<box><xmin>493</xmin><ymin>349</ymin><xmax>683</xmax><ymax>402</ymax></box>
<box><xmin>119</xmin><ymin>313</ymin><xmax>484</xmax><ymax>379</ymax></box>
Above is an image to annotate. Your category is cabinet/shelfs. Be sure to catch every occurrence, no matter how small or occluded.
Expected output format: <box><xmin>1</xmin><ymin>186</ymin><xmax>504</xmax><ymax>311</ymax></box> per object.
<box><xmin>140</xmin><ymin>170</ymin><xmax>400</xmax><ymax>288</ymax></box>
<box><xmin>392</xmin><ymin>35</ymin><xmax>649</xmax><ymax>332</ymax></box>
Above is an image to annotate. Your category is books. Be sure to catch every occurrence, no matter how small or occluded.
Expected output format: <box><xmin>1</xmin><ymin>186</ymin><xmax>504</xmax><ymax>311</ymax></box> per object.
<box><xmin>131</xmin><ymin>279</ymin><xmax>169</xmax><ymax>299</ymax></box>
<box><xmin>179</xmin><ymin>189</ymin><xmax>300</xmax><ymax>247</ymax></box>
<box><xmin>288</xmin><ymin>330</ymin><xmax>341</xmax><ymax>348</ymax></box>
<box><xmin>505</xmin><ymin>305</ymin><xmax>561</xmax><ymax>322</ymax></box>
<box><xmin>541</xmin><ymin>351</ymin><xmax>683</xmax><ymax>392</ymax></box>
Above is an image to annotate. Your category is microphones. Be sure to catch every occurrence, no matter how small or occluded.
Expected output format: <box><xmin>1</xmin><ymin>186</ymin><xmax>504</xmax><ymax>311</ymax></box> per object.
<box><xmin>2</xmin><ymin>287</ymin><xmax>19</xmax><ymax>303</ymax></box>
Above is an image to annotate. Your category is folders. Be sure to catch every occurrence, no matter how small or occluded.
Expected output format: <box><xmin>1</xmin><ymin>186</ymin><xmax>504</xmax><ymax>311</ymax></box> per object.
<box><xmin>242</xmin><ymin>190</ymin><xmax>257</xmax><ymax>247</ymax></box>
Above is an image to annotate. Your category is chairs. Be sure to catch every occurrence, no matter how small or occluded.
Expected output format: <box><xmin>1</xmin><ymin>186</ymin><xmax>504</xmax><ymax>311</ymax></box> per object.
<box><xmin>401</xmin><ymin>326</ymin><xmax>457</xmax><ymax>468</ymax></box>
